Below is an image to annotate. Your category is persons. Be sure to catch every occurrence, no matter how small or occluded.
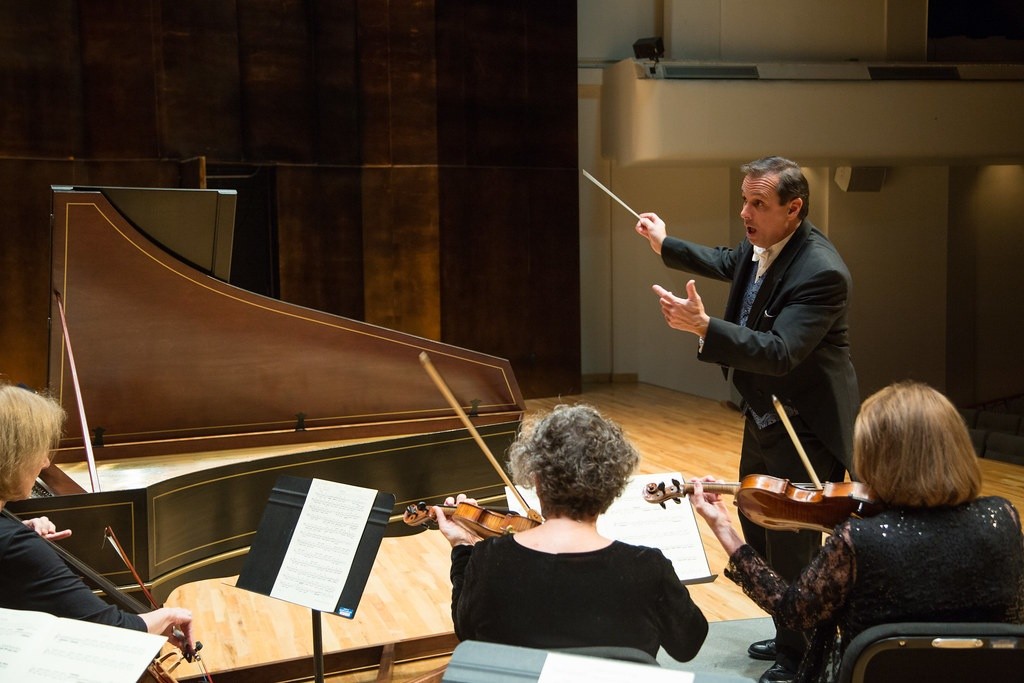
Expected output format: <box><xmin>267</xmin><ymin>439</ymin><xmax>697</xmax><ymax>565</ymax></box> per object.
<box><xmin>636</xmin><ymin>156</ymin><xmax>859</xmax><ymax>683</ymax></box>
<box><xmin>691</xmin><ymin>380</ymin><xmax>1024</xmax><ymax>683</ymax></box>
<box><xmin>432</xmin><ymin>403</ymin><xmax>709</xmax><ymax>664</ymax></box>
<box><xmin>1</xmin><ymin>385</ymin><xmax>194</xmax><ymax>663</ymax></box>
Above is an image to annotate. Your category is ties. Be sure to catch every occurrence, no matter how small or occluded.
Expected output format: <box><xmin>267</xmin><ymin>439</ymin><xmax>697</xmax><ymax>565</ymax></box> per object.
<box><xmin>752</xmin><ymin>245</ymin><xmax>769</xmax><ymax>268</ymax></box>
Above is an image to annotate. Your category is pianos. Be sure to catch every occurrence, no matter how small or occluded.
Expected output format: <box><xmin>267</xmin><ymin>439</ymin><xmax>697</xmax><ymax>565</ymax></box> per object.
<box><xmin>6</xmin><ymin>182</ymin><xmax>529</xmax><ymax>612</ymax></box>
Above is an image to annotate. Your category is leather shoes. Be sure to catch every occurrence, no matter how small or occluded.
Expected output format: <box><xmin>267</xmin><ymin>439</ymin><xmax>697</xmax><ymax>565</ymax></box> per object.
<box><xmin>748</xmin><ymin>638</ymin><xmax>777</xmax><ymax>660</ymax></box>
<box><xmin>759</xmin><ymin>662</ymin><xmax>798</xmax><ymax>683</ymax></box>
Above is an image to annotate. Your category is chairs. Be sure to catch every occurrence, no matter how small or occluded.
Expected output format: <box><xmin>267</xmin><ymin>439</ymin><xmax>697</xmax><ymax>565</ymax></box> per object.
<box><xmin>839</xmin><ymin>622</ymin><xmax>1024</xmax><ymax>683</ymax></box>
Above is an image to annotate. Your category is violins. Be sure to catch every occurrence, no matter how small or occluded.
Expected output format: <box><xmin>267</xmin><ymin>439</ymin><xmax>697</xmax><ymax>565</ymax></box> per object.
<box><xmin>387</xmin><ymin>497</ymin><xmax>541</xmax><ymax>541</ymax></box>
<box><xmin>640</xmin><ymin>473</ymin><xmax>874</xmax><ymax>533</ymax></box>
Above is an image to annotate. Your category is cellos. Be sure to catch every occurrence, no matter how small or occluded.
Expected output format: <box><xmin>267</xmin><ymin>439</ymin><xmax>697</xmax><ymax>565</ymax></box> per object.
<box><xmin>1</xmin><ymin>509</ymin><xmax>184</xmax><ymax>683</ymax></box>
